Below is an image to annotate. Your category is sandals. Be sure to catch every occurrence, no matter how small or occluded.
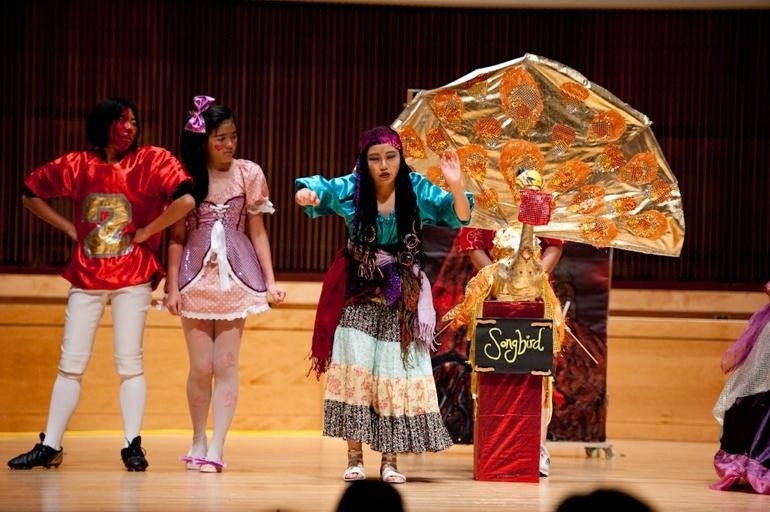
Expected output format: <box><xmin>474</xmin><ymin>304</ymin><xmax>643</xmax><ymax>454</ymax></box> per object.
<box><xmin>380</xmin><ymin>451</ymin><xmax>408</xmax><ymax>486</ymax></box>
<box><xmin>342</xmin><ymin>448</ymin><xmax>368</xmax><ymax>481</ymax></box>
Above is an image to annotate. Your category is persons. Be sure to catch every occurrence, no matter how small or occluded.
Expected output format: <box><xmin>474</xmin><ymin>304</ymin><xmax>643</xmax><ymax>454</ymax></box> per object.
<box><xmin>712</xmin><ymin>275</ymin><xmax>769</xmax><ymax>496</ymax></box>
<box><xmin>6</xmin><ymin>94</ymin><xmax>199</xmax><ymax>471</ymax></box>
<box><xmin>294</xmin><ymin>127</ymin><xmax>475</xmax><ymax>483</ymax></box>
<box><xmin>428</xmin><ymin>224</ymin><xmax>607</xmax><ymax>440</ymax></box>
<box><xmin>163</xmin><ymin>96</ymin><xmax>286</xmax><ymax>474</ymax></box>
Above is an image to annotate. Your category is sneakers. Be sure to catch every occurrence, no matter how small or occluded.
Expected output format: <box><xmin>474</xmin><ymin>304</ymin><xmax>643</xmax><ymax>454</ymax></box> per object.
<box><xmin>538</xmin><ymin>444</ymin><xmax>552</xmax><ymax>477</ymax></box>
<box><xmin>198</xmin><ymin>460</ymin><xmax>224</xmax><ymax>474</ymax></box>
<box><xmin>7</xmin><ymin>442</ymin><xmax>64</xmax><ymax>470</ymax></box>
<box><xmin>185</xmin><ymin>456</ymin><xmax>202</xmax><ymax>473</ymax></box>
<box><xmin>120</xmin><ymin>435</ymin><xmax>149</xmax><ymax>472</ymax></box>
